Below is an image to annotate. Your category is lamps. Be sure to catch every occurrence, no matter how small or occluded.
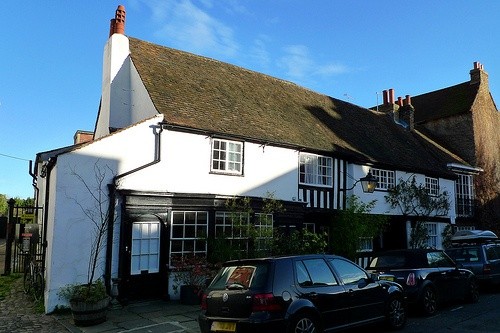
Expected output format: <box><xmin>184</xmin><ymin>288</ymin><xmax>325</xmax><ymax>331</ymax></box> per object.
<box><xmin>340</xmin><ymin>169</ymin><xmax>379</xmax><ymax>194</ymax></box>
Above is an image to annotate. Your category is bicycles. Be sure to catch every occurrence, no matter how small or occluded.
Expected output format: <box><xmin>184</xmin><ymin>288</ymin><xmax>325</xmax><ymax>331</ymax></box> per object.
<box><xmin>16</xmin><ymin>252</ymin><xmax>45</xmax><ymax>302</ymax></box>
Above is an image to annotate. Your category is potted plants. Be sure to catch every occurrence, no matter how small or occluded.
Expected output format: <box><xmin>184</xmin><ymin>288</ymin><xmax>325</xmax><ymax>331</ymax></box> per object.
<box><xmin>57</xmin><ymin>277</ymin><xmax>111</xmax><ymax>326</ymax></box>
<box><xmin>168</xmin><ymin>255</ymin><xmax>223</xmax><ymax>305</ymax></box>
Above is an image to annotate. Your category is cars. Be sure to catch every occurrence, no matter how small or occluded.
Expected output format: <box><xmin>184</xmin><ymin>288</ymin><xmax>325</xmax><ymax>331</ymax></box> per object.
<box><xmin>440</xmin><ymin>231</ymin><xmax>500</xmax><ymax>284</ymax></box>
<box><xmin>364</xmin><ymin>248</ymin><xmax>481</xmax><ymax>316</ymax></box>
<box><xmin>199</xmin><ymin>252</ymin><xmax>407</xmax><ymax>332</ymax></box>
